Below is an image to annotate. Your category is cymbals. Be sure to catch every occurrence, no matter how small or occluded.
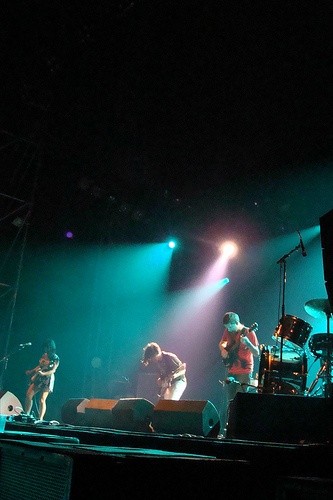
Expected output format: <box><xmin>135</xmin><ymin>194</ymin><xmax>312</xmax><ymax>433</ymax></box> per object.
<box><xmin>304</xmin><ymin>299</ymin><xmax>333</xmax><ymax>324</ymax></box>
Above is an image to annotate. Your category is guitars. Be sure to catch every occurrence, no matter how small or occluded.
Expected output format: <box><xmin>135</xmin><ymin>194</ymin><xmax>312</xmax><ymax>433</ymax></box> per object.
<box><xmin>26</xmin><ymin>359</ymin><xmax>57</xmax><ymax>382</ymax></box>
<box><xmin>220</xmin><ymin>322</ymin><xmax>259</xmax><ymax>368</ymax></box>
<box><xmin>155</xmin><ymin>362</ymin><xmax>186</xmax><ymax>397</ymax></box>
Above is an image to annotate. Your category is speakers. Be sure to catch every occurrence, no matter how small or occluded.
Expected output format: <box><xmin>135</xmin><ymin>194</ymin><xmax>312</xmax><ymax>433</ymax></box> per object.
<box><xmin>61</xmin><ymin>397</ymin><xmax>221</xmax><ymax>438</ymax></box>
<box><xmin>0</xmin><ymin>390</ymin><xmax>23</xmax><ymax>416</ymax></box>
<box><xmin>226</xmin><ymin>392</ymin><xmax>333</xmax><ymax>445</ymax></box>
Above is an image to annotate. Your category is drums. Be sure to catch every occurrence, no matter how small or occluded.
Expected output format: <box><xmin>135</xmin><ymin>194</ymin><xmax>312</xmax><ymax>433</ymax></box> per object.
<box><xmin>308</xmin><ymin>333</ymin><xmax>333</xmax><ymax>360</ymax></box>
<box><xmin>271</xmin><ymin>314</ymin><xmax>313</xmax><ymax>350</ymax></box>
<box><xmin>258</xmin><ymin>345</ymin><xmax>307</xmax><ymax>395</ymax></box>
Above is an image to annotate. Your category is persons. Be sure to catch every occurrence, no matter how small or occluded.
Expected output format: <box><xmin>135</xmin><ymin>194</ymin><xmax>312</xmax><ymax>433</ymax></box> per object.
<box><xmin>24</xmin><ymin>339</ymin><xmax>60</xmax><ymax>424</ymax></box>
<box><xmin>216</xmin><ymin>311</ymin><xmax>259</xmax><ymax>439</ymax></box>
<box><xmin>142</xmin><ymin>343</ymin><xmax>188</xmax><ymax>401</ymax></box>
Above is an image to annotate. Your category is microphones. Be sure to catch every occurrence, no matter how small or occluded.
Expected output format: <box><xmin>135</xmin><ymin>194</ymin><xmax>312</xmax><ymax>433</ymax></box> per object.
<box><xmin>298</xmin><ymin>232</ymin><xmax>306</xmax><ymax>256</ymax></box>
<box><xmin>20</xmin><ymin>342</ymin><xmax>32</xmax><ymax>346</ymax></box>
<box><xmin>225</xmin><ymin>376</ymin><xmax>235</xmax><ymax>384</ymax></box>
